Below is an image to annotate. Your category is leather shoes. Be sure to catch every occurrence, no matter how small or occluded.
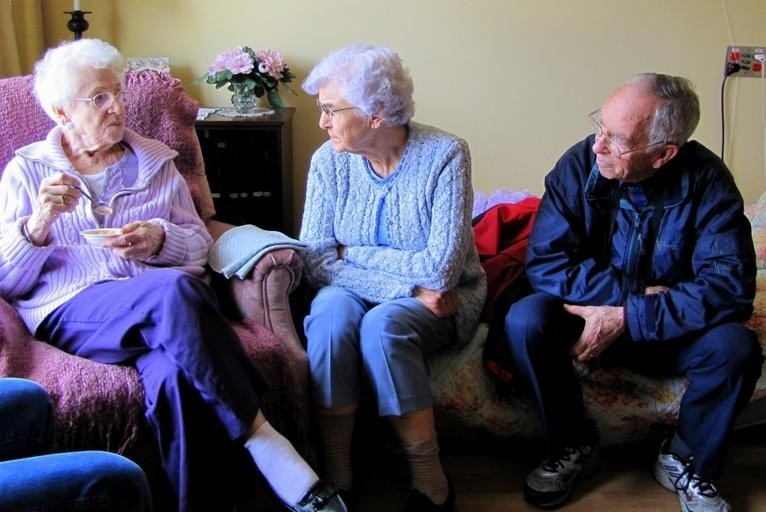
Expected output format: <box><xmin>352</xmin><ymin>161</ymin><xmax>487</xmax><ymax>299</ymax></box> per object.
<box><xmin>264</xmin><ymin>477</ymin><xmax>348</xmax><ymax>512</ymax></box>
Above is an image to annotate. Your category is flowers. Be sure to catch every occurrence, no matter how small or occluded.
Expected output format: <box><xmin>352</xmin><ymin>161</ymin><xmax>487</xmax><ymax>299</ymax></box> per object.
<box><xmin>191</xmin><ymin>45</ymin><xmax>299</xmax><ymax>112</ymax></box>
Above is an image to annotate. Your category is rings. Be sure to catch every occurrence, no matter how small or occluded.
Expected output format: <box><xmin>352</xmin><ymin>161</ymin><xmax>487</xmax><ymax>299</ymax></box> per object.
<box><xmin>60</xmin><ymin>197</ymin><xmax>66</xmax><ymax>203</ymax></box>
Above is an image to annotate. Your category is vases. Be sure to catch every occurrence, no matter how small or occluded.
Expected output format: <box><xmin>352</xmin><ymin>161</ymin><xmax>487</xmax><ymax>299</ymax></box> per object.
<box><xmin>230</xmin><ymin>82</ymin><xmax>258</xmax><ymax>114</ymax></box>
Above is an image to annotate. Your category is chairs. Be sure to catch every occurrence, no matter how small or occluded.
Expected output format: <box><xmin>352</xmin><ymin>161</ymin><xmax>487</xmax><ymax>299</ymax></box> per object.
<box><xmin>0</xmin><ymin>65</ymin><xmax>310</xmax><ymax>512</ymax></box>
<box><xmin>424</xmin><ymin>191</ymin><xmax>766</xmax><ymax>445</ymax></box>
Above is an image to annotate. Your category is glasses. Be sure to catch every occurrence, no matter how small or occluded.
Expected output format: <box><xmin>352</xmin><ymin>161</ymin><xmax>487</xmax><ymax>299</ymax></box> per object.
<box><xmin>73</xmin><ymin>89</ymin><xmax>132</xmax><ymax>109</ymax></box>
<box><xmin>315</xmin><ymin>99</ymin><xmax>355</xmax><ymax>119</ymax></box>
<box><xmin>587</xmin><ymin>108</ymin><xmax>663</xmax><ymax>159</ymax></box>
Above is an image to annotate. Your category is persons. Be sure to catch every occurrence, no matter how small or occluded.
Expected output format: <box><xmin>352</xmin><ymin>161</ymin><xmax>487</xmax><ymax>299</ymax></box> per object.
<box><xmin>295</xmin><ymin>41</ymin><xmax>486</xmax><ymax>512</ymax></box>
<box><xmin>504</xmin><ymin>73</ymin><xmax>763</xmax><ymax>512</ymax></box>
<box><xmin>0</xmin><ymin>377</ymin><xmax>150</xmax><ymax>512</ymax></box>
<box><xmin>0</xmin><ymin>36</ymin><xmax>356</xmax><ymax>511</ymax></box>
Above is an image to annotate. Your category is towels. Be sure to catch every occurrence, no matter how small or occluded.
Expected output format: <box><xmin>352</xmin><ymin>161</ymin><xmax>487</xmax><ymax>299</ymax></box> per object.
<box><xmin>205</xmin><ymin>223</ymin><xmax>313</xmax><ymax>283</ymax></box>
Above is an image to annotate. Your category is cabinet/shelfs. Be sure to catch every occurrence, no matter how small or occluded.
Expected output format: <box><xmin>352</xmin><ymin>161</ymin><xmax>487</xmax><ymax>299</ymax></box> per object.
<box><xmin>193</xmin><ymin>105</ymin><xmax>298</xmax><ymax>232</ymax></box>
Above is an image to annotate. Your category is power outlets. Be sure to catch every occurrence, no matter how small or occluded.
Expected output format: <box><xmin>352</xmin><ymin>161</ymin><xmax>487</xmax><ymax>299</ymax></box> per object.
<box><xmin>721</xmin><ymin>45</ymin><xmax>766</xmax><ymax>79</ymax></box>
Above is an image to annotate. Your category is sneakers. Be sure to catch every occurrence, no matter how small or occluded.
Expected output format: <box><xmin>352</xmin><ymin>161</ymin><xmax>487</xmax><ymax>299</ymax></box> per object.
<box><xmin>655</xmin><ymin>438</ymin><xmax>732</xmax><ymax>511</ymax></box>
<box><xmin>407</xmin><ymin>483</ymin><xmax>454</xmax><ymax>511</ymax></box>
<box><xmin>523</xmin><ymin>447</ymin><xmax>600</xmax><ymax>508</ymax></box>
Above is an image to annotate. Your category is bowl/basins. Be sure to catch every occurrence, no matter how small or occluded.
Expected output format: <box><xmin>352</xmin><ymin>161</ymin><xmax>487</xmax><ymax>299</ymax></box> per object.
<box><xmin>81</xmin><ymin>228</ymin><xmax>123</xmax><ymax>247</ymax></box>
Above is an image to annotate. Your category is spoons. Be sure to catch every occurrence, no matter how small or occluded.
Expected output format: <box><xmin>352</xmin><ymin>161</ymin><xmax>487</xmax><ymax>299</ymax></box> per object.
<box><xmin>72</xmin><ymin>184</ymin><xmax>113</xmax><ymax>216</ymax></box>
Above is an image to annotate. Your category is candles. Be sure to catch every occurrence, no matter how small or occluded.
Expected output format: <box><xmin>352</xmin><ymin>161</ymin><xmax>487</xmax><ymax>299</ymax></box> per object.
<box><xmin>73</xmin><ymin>0</ymin><xmax>80</xmax><ymax>9</ymax></box>
